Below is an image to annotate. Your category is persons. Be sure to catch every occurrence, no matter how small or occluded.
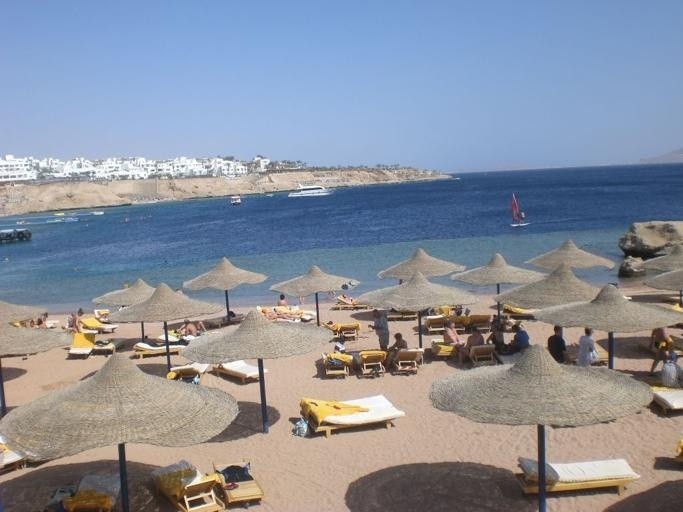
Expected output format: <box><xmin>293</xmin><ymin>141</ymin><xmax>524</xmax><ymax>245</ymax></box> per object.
<box><xmin>519</xmin><ymin>210</ymin><xmax>525</xmax><ymax>224</ymax></box>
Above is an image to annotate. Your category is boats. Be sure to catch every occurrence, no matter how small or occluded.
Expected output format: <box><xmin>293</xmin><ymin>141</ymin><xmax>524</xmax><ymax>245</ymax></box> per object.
<box><xmin>230</xmin><ymin>195</ymin><xmax>241</xmax><ymax>205</ymax></box>
<box><xmin>510</xmin><ymin>191</ymin><xmax>530</xmax><ymax>229</ymax></box>
<box><xmin>287</xmin><ymin>183</ymin><xmax>333</xmax><ymax>198</ymax></box>
